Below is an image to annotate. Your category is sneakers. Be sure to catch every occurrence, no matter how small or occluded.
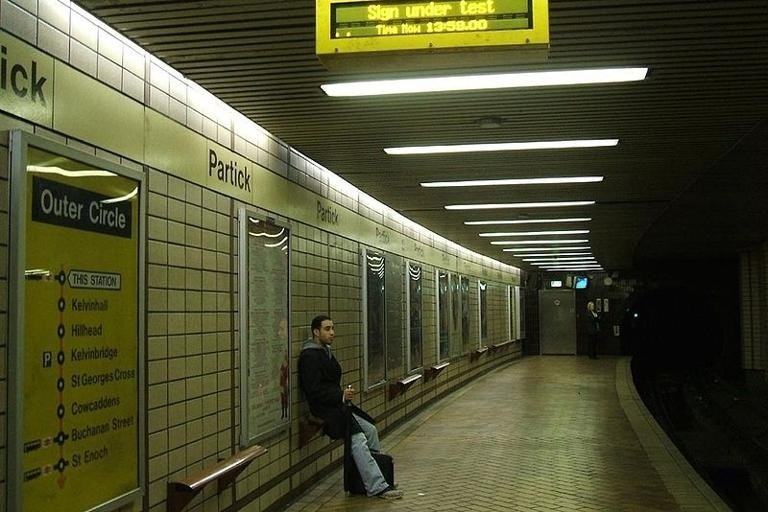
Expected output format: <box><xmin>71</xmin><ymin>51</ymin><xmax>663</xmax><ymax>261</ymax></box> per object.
<box><xmin>377</xmin><ymin>487</ymin><xmax>403</xmax><ymax>499</ymax></box>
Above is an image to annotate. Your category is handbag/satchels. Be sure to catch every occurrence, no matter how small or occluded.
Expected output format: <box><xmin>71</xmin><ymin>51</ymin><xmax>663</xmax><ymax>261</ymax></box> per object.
<box><xmin>343</xmin><ymin>452</ymin><xmax>393</xmax><ymax>493</ymax></box>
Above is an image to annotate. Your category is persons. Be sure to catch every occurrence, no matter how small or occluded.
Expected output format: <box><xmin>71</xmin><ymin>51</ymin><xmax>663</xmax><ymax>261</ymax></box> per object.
<box><xmin>584</xmin><ymin>301</ymin><xmax>599</xmax><ymax>360</ymax></box>
<box><xmin>299</xmin><ymin>316</ymin><xmax>403</xmax><ymax>500</ymax></box>
